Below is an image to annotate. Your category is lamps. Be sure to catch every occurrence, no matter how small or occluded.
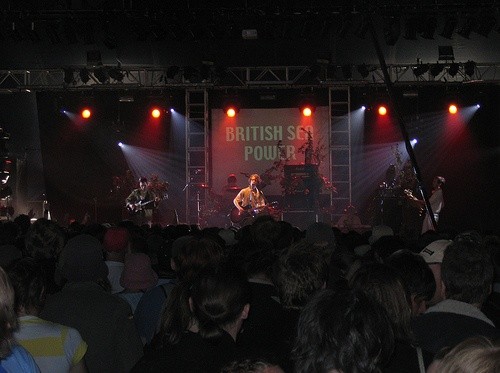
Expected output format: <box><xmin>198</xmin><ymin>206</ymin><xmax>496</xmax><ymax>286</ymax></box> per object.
<box><xmin>413</xmin><ymin>60</ymin><xmax>475</xmax><ymax>77</ymax></box>
<box><xmin>63</xmin><ymin>66</ymin><xmax>123</xmax><ymax>83</ymax></box>
<box><xmin>308</xmin><ymin>63</ymin><xmax>371</xmax><ymax>80</ymax></box>
<box><xmin>82</xmin><ymin>100</ymin><xmax>95</xmax><ymax>119</ymax></box>
<box><xmin>222</xmin><ymin>93</ymin><xmax>242</xmax><ymax>119</ymax></box>
<box><xmin>300</xmin><ymin>96</ymin><xmax>316</xmax><ymax>118</ymax></box>
<box><xmin>149</xmin><ymin>95</ymin><xmax>175</xmax><ymax>119</ymax></box>
<box><xmin>376</xmin><ymin>96</ymin><xmax>389</xmax><ymax>116</ymax></box>
<box><xmin>447</xmin><ymin>99</ymin><xmax>459</xmax><ymax>115</ymax></box>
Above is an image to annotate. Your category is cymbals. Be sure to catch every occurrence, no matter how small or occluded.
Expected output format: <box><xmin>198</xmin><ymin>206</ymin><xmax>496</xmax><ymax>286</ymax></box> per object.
<box><xmin>226</xmin><ymin>187</ymin><xmax>242</xmax><ymax>193</ymax></box>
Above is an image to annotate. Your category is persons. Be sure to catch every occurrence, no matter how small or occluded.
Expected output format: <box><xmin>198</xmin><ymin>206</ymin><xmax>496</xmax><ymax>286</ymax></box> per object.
<box><xmin>0</xmin><ymin>214</ymin><xmax>500</xmax><ymax>373</ymax></box>
<box><xmin>219</xmin><ymin>175</ymin><xmax>239</xmax><ymax>196</ymax></box>
<box><xmin>232</xmin><ymin>174</ymin><xmax>266</xmax><ymax>228</ymax></box>
<box><xmin>407</xmin><ymin>177</ymin><xmax>446</xmax><ymax>234</ymax></box>
<box><xmin>124</xmin><ymin>177</ymin><xmax>161</xmax><ymax>228</ymax></box>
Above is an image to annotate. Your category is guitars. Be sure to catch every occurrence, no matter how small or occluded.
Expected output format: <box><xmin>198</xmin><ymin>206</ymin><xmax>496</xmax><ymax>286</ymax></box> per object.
<box><xmin>125</xmin><ymin>193</ymin><xmax>169</xmax><ymax>215</ymax></box>
<box><xmin>229</xmin><ymin>201</ymin><xmax>279</xmax><ymax>223</ymax></box>
<box><xmin>404</xmin><ymin>189</ymin><xmax>427</xmax><ymax>220</ymax></box>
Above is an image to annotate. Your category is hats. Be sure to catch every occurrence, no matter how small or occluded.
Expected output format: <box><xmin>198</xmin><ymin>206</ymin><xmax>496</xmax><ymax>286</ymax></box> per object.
<box><xmin>60</xmin><ymin>235</ymin><xmax>109</xmax><ymax>280</ymax></box>
<box><xmin>118</xmin><ymin>252</ymin><xmax>159</xmax><ymax>291</ymax></box>
<box><xmin>369</xmin><ymin>225</ymin><xmax>394</xmax><ymax>245</ymax></box>
<box><xmin>420</xmin><ymin>239</ymin><xmax>454</xmax><ymax>264</ymax></box>
<box><xmin>104</xmin><ymin>227</ymin><xmax>129</xmax><ymax>252</ymax></box>
<box><xmin>305</xmin><ymin>222</ymin><xmax>335</xmax><ymax>250</ymax></box>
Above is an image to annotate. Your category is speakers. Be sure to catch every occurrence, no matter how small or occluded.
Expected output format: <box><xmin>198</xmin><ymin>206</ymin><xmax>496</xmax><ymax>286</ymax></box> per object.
<box><xmin>381</xmin><ymin>198</ymin><xmax>421</xmax><ymax>240</ymax></box>
<box><xmin>281</xmin><ymin>164</ymin><xmax>320</xmax><ymax>228</ymax></box>
